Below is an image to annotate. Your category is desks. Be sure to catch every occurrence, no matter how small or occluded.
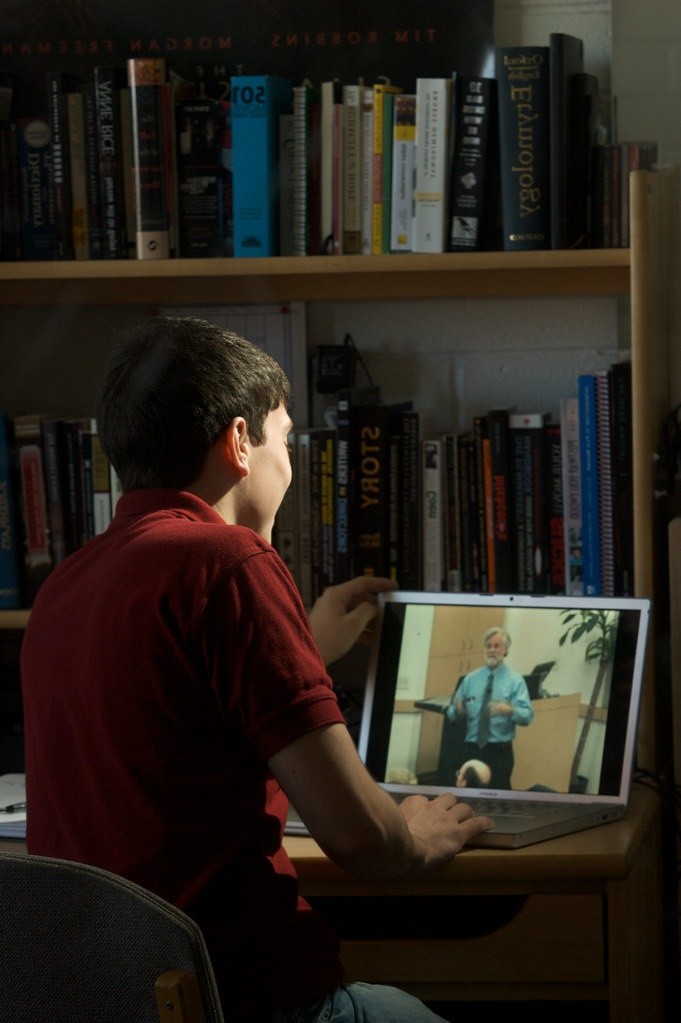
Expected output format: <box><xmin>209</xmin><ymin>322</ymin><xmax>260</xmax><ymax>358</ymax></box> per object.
<box><xmin>0</xmin><ymin>772</ymin><xmax>664</xmax><ymax>1023</ymax></box>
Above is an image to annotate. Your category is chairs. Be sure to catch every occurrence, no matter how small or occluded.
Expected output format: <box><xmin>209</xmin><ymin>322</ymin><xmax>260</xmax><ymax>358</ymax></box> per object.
<box><xmin>0</xmin><ymin>855</ymin><xmax>226</xmax><ymax>1023</ymax></box>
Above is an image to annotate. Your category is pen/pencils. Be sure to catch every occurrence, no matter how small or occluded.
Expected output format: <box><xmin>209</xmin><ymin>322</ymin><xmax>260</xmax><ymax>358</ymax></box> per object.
<box><xmin>6</xmin><ymin>803</ymin><xmax>26</xmax><ymax>813</ymax></box>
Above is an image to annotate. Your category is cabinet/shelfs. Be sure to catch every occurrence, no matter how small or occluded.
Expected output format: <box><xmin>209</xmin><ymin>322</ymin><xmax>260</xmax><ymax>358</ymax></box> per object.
<box><xmin>1</xmin><ymin>167</ymin><xmax>681</xmax><ymax>778</ymax></box>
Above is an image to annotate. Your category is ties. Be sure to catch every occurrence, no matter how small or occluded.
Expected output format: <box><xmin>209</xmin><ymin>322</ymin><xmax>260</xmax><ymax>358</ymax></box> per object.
<box><xmin>478</xmin><ymin>673</ymin><xmax>495</xmax><ymax>750</ymax></box>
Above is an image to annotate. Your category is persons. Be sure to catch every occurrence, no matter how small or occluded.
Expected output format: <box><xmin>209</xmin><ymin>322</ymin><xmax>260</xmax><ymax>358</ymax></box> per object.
<box><xmin>447</xmin><ymin>626</ymin><xmax>534</xmax><ymax>791</ymax></box>
<box><xmin>456</xmin><ymin>759</ymin><xmax>491</xmax><ymax>787</ymax></box>
<box><xmin>19</xmin><ymin>315</ymin><xmax>496</xmax><ymax>1023</ymax></box>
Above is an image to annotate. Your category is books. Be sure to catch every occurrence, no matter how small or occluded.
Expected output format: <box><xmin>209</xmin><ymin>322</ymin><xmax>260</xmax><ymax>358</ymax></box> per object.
<box><xmin>0</xmin><ymin>360</ymin><xmax>632</xmax><ymax>610</ymax></box>
<box><xmin>0</xmin><ymin>33</ymin><xmax>659</xmax><ymax>262</ymax></box>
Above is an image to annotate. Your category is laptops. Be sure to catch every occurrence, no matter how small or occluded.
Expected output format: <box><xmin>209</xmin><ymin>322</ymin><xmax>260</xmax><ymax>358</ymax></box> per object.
<box><xmin>531</xmin><ymin>661</ymin><xmax>556</xmax><ymax>685</ymax></box>
<box><xmin>283</xmin><ymin>589</ymin><xmax>651</xmax><ymax>851</ymax></box>
